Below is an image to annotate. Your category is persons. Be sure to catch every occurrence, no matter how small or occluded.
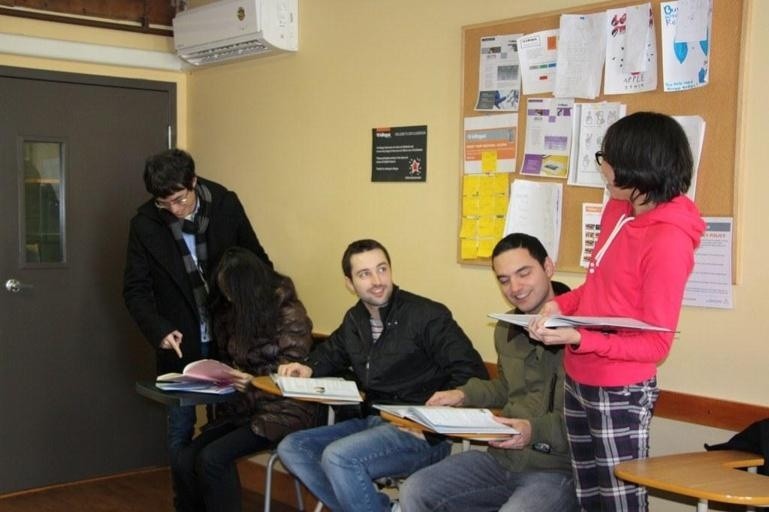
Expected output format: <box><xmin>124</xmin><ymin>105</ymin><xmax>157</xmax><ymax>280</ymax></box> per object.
<box><xmin>124</xmin><ymin>148</ymin><xmax>275</xmax><ymax>511</ymax></box>
<box><xmin>399</xmin><ymin>233</ymin><xmax>580</xmax><ymax>511</ymax></box>
<box><xmin>276</xmin><ymin>239</ymin><xmax>491</xmax><ymax>511</ymax></box>
<box><xmin>523</xmin><ymin>112</ymin><xmax>706</xmax><ymax>510</ymax></box>
<box><xmin>174</xmin><ymin>247</ymin><xmax>319</xmax><ymax>512</ymax></box>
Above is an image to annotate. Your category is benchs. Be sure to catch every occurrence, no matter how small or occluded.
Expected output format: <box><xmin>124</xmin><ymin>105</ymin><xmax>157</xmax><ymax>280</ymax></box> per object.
<box><xmin>240</xmin><ymin>331</ymin><xmax>509</xmax><ymax>512</ymax></box>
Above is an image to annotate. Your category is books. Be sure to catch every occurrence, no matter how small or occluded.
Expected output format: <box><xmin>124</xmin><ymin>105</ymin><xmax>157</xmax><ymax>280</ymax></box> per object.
<box><xmin>156</xmin><ymin>359</ymin><xmax>245</xmax><ymax>386</ymax></box>
<box><xmin>489</xmin><ymin>313</ymin><xmax>671</xmax><ymax>332</ymax></box>
<box><xmin>372</xmin><ymin>404</ymin><xmax>521</xmax><ymax>435</ymax></box>
<box><xmin>270</xmin><ymin>372</ymin><xmax>364</xmax><ymax>402</ymax></box>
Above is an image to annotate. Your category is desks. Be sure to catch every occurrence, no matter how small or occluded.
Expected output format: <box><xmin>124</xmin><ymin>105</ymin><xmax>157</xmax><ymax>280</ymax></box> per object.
<box><xmin>136</xmin><ymin>376</ymin><xmax>241</xmax><ymax>465</ymax></box>
<box><xmin>249</xmin><ymin>375</ymin><xmax>512</xmax><ymax>512</ymax></box>
<box><xmin>608</xmin><ymin>447</ymin><xmax>769</xmax><ymax>512</ymax></box>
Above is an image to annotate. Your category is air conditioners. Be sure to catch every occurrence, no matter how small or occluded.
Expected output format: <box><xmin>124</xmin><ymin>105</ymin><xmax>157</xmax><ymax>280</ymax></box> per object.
<box><xmin>170</xmin><ymin>0</ymin><xmax>299</xmax><ymax>69</ymax></box>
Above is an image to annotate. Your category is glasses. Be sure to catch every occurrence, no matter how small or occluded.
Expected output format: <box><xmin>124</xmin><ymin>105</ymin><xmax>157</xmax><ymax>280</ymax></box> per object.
<box><xmin>595</xmin><ymin>150</ymin><xmax>606</xmax><ymax>165</ymax></box>
<box><xmin>154</xmin><ymin>192</ymin><xmax>189</xmax><ymax>208</ymax></box>
